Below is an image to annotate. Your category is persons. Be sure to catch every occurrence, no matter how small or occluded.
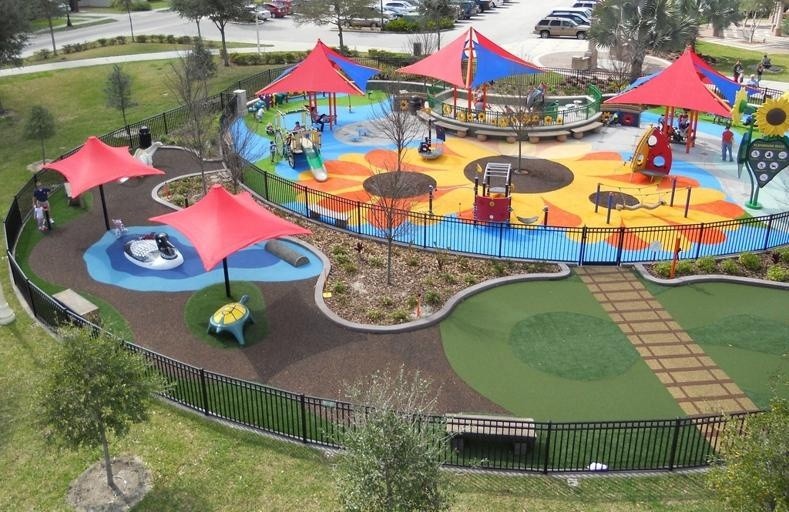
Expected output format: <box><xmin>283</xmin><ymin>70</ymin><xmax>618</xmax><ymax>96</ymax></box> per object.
<box><xmin>31</xmin><ymin>180</ymin><xmax>55</xmax><ymax>225</ymax></box>
<box><xmin>473</xmin><ymin>80</ymin><xmax>546</xmax><ymax>118</ymax></box>
<box><xmin>252</xmin><ymin>93</ymin><xmax>326</xmax><ymax>164</ymax></box>
<box><xmin>33</xmin><ymin>200</ymin><xmax>48</xmax><ymax>233</ymax></box>
<box><xmin>720</xmin><ymin>123</ymin><xmax>734</xmax><ymax>162</ymax></box>
<box><xmin>657</xmin><ymin>54</ymin><xmax>771</xmax><ymax>142</ymax></box>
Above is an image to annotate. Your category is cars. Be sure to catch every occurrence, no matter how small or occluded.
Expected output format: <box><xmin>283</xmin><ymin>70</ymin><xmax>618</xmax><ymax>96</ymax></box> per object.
<box><xmin>535</xmin><ymin>1</ymin><xmax>599</xmax><ymax>40</ymax></box>
<box><xmin>446</xmin><ymin>0</ymin><xmax>503</xmax><ymax>22</ymax></box>
<box><xmin>245</xmin><ymin>0</ymin><xmax>291</xmax><ymax>21</ymax></box>
<box><xmin>335</xmin><ymin>0</ymin><xmax>423</xmax><ymax>30</ymax></box>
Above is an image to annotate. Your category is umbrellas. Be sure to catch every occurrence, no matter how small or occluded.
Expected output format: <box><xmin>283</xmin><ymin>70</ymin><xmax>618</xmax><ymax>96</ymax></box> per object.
<box><xmin>41</xmin><ymin>134</ymin><xmax>166</xmax><ymax>232</ymax></box>
<box><xmin>146</xmin><ymin>182</ymin><xmax>314</xmax><ymax>295</ymax></box>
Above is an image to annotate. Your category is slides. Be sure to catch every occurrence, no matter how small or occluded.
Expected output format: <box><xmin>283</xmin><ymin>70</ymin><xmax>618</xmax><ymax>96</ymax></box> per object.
<box><xmin>301</xmin><ymin>145</ymin><xmax>328</xmax><ymax>182</ymax></box>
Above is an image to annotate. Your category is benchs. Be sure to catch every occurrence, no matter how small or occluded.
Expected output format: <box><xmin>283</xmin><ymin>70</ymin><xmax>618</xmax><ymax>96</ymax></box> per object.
<box><xmin>52</xmin><ymin>288</ymin><xmax>100</xmax><ymax>331</ymax></box>
<box><xmin>446</xmin><ymin>413</ymin><xmax>537</xmax><ymax>455</ymax></box>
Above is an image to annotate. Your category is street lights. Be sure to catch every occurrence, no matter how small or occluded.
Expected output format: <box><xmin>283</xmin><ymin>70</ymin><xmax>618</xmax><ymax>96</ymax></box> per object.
<box><xmin>252</xmin><ymin>3</ymin><xmax>261</xmax><ymax>55</ymax></box>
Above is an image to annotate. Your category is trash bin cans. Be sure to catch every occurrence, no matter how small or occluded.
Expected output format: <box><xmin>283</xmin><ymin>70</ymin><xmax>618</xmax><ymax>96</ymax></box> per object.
<box><xmin>409</xmin><ymin>95</ymin><xmax>421</xmax><ymax>116</ymax></box>
<box><xmin>413</xmin><ymin>41</ymin><xmax>422</xmax><ymax>57</ymax></box>
<box><xmin>138</xmin><ymin>125</ymin><xmax>152</xmax><ymax>149</ymax></box>
